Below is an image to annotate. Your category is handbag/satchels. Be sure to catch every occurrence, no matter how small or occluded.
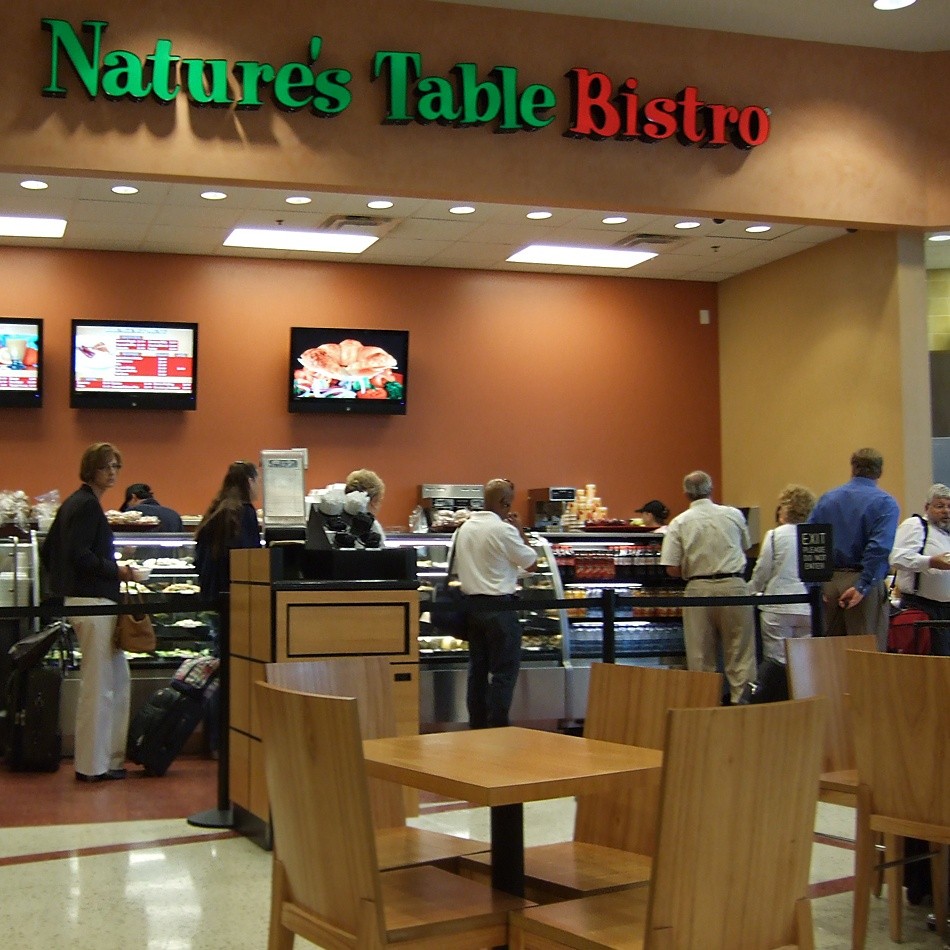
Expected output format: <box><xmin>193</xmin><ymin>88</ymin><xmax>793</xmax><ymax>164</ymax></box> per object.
<box><xmin>885</xmin><ymin>601</ymin><xmax>932</xmax><ymax>654</ymax></box>
<box><xmin>111</xmin><ymin>564</ymin><xmax>157</xmax><ymax>652</ymax></box>
<box><xmin>432</xmin><ymin>582</ymin><xmax>480</xmax><ymax>642</ymax></box>
<box><xmin>8</xmin><ymin>624</ymin><xmax>62</xmax><ymax>665</ymax></box>
<box><xmin>168</xmin><ymin>652</ymin><xmax>223</xmax><ymax>702</ymax></box>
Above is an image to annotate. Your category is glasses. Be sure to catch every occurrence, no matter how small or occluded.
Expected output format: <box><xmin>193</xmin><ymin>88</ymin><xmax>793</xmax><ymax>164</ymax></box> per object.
<box><xmin>96</xmin><ymin>463</ymin><xmax>123</xmax><ymax>471</ymax></box>
<box><xmin>934</xmin><ymin>503</ymin><xmax>950</xmax><ymax>510</ymax></box>
<box><xmin>504</xmin><ymin>478</ymin><xmax>514</xmax><ymax>490</ymax></box>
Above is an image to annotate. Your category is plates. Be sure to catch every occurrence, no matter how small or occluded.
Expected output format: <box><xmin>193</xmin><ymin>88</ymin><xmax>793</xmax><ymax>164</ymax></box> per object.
<box><xmin>115</xmin><ymin>552</ymin><xmax>198</xmax><ymax>593</ymax></box>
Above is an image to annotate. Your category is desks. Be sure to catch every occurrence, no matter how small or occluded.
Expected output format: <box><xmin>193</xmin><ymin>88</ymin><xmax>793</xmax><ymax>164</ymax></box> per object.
<box><xmin>362</xmin><ymin>726</ymin><xmax>664</xmax><ymax>899</ymax></box>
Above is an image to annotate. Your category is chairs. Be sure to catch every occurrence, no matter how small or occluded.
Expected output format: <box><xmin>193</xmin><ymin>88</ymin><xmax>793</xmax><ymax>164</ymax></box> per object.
<box><xmin>255</xmin><ymin>634</ymin><xmax>950</xmax><ymax>950</ymax></box>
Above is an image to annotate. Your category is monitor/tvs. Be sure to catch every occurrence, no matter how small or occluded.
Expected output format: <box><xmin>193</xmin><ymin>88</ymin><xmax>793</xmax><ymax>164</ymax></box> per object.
<box><xmin>69</xmin><ymin>317</ymin><xmax>199</xmax><ymax>410</ymax></box>
<box><xmin>287</xmin><ymin>326</ymin><xmax>410</xmax><ymax>415</ymax></box>
<box><xmin>0</xmin><ymin>316</ymin><xmax>43</xmax><ymax>409</ymax></box>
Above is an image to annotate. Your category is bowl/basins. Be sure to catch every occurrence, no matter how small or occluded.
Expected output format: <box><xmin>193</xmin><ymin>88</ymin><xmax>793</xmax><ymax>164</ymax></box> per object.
<box><xmin>131</xmin><ymin>566</ymin><xmax>153</xmax><ymax>581</ymax></box>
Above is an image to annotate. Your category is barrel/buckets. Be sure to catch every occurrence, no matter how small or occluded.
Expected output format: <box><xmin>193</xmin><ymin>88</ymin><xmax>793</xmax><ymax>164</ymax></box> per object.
<box><xmin>0</xmin><ymin>571</ymin><xmax>30</xmax><ymax>607</ymax></box>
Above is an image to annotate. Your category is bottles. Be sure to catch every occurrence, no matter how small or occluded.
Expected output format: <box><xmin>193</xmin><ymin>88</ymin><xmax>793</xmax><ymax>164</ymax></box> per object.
<box><xmin>548</xmin><ymin>540</ymin><xmax>687</xmax><ymax>656</ymax></box>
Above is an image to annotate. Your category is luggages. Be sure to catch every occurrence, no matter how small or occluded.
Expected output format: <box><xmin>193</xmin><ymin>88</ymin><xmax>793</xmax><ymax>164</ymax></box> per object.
<box><xmin>124</xmin><ymin>687</ymin><xmax>203</xmax><ymax>776</ymax></box>
<box><xmin>2</xmin><ymin>666</ymin><xmax>63</xmax><ymax>775</ymax></box>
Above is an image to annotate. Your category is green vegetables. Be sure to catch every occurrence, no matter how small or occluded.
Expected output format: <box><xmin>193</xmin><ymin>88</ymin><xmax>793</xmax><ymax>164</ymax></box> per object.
<box><xmin>291</xmin><ymin>377</ymin><xmax>402</xmax><ymax>400</ymax></box>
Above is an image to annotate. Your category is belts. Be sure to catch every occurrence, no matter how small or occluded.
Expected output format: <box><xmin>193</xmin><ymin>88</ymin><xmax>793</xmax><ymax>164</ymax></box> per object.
<box><xmin>835</xmin><ymin>565</ymin><xmax>865</xmax><ymax>572</ymax></box>
<box><xmin>686</xmin><ymin>573</ymin><xmax>743</xmax><ymax>582</ymax></box>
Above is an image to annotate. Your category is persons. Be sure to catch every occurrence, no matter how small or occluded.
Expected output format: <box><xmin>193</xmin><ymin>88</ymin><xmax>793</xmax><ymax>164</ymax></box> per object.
<box><xmin>446</xmin><ymin>478</ymin><xmax>539</xmax><ymax>728</ymax></box>
<box><xmin>43</xmin><ymin>443</ymin><xmax>140</xmax><ymax>782</ymax></box>
<box><xmin>115</xmin><ymin>482</ymin><xmax>184</xmax><ymax>532</ymax></box>
<box><xmin>197</xmin><ymin>461</ymin><xmax>264</xmax><ymax>760</ymax></box>
<box><xmin>801</xmin><ymin>449</ymin><xmax>900</xmax><ymax>653</ymax></box>
<box><xmin>634</xmin><ymin>500</ymin><xmax>671</xmax><ymax>536</ymax></box>
<box><xmin>890</xmin><ymin>483</ymin><xmax>950</xmax><ymax>653</ymax></box>
<box><xmin>751</xmin><ymin>484</ymin><xmax>815</xmax><ymax>702</ymax></box>
<box><xmin>340</xmin><ymin>468</ymin><xmax>389</xmax><ymax>549</ymax></box>
<box><xmin>661</xmin><ymin>471</ymin><xmax>758</xmax><ymax>706</ymax></box>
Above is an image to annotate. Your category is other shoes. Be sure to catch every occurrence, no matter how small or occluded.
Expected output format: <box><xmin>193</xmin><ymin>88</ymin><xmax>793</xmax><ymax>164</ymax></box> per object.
<box><xmin>75</xmin><ymin>769</ymin><xmax>126</xmax><ymax>781</ymax></box>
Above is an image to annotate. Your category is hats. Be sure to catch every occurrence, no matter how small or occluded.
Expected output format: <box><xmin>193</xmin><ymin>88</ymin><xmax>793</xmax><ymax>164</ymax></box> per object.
<box><xmin>118</xmin><ymin>484</ymin><xmax>153</xmax><ymax>511</ymax></box>
<box><xmin>634</xmin><ymin>500</ymin><xmax>667</xmax><ymax>519</ymax></box>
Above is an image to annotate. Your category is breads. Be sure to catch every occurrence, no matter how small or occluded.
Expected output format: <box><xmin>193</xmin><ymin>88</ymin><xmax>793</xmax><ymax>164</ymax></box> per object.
<box><xmin>298</xmin><ymin>339</ymin><xmax>395</xmax><ymax>379</ymax></box>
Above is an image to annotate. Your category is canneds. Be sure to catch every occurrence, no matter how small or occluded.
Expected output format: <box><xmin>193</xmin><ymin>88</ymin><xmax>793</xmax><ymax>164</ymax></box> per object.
<box><xmin>631</xmin><ymin>589</ymin><xmax>685</xmax><ymax>617</ymax></box>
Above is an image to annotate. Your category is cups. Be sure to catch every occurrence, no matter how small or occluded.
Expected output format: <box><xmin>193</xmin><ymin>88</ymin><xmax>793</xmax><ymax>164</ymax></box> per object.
<box><xmin>6</xmin><ymin>338</ymin><xmax>26</xmax><ymax>362</ymax></box>
<box><xmin>566</xmin><ymin>482</ymin><xmax>610</xmax><ymax>523</ymax></box>
<box><xmin>23</xmin><ymin>348</ymin><xmax>38</xmax><ymax>366</ymax></box>
